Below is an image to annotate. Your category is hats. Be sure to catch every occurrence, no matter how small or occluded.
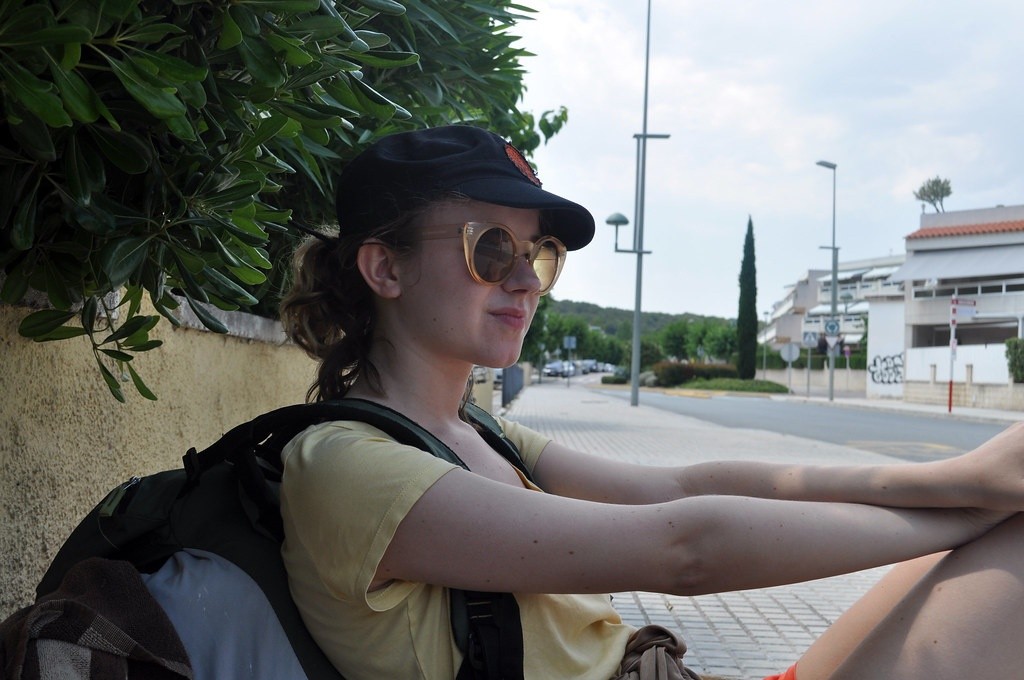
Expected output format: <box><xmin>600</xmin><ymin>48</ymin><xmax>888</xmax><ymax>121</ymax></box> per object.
<box><xmin>334</xmin><ymin>125</ymin><xmax>595</xmax><ymax>251</ymax></box>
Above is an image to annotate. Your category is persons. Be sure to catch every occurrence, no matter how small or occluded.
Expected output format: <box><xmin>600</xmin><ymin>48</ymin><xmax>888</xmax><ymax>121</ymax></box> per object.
<box><xmin>274</xmin><ymin>127</ymin><xmax>1024</xmax><ymax>680</ymax></box>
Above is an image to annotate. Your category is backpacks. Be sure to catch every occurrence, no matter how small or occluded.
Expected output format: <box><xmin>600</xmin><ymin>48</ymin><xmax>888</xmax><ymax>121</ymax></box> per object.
<box><xmin>0</xmin><ymin>399</ymin><xmax>534</xmax><ymax>680</ymax></box>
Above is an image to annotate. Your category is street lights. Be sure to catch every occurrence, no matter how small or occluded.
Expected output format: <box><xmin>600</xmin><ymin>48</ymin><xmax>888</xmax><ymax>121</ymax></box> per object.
<box><xmin>816</xmin><ymin>161</ymin><xmax>840</xmax><ymax>400</ymax></box>
<box><xmin>606</xmin><ymin>214</ymin><xmax>651</xmax><ymax>407</ymax></box>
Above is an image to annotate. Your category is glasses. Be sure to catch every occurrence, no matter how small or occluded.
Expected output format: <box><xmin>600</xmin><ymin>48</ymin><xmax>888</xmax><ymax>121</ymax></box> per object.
<box><xmin>403</xmin><ymin>219</ymin><xmax>567</xmax><ymax>296</ymax></box>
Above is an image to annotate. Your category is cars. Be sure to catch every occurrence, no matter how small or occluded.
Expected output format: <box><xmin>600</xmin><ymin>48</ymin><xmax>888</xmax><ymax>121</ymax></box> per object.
<box><xmin>543</xmin><ymin>359</ymin><xmax>613</xmax><ymax>377</ymax></box>
<box><xmin>471</xmin><ymin>364</ymin><xmax>503</xmax><ymax>384</ymax></box>
